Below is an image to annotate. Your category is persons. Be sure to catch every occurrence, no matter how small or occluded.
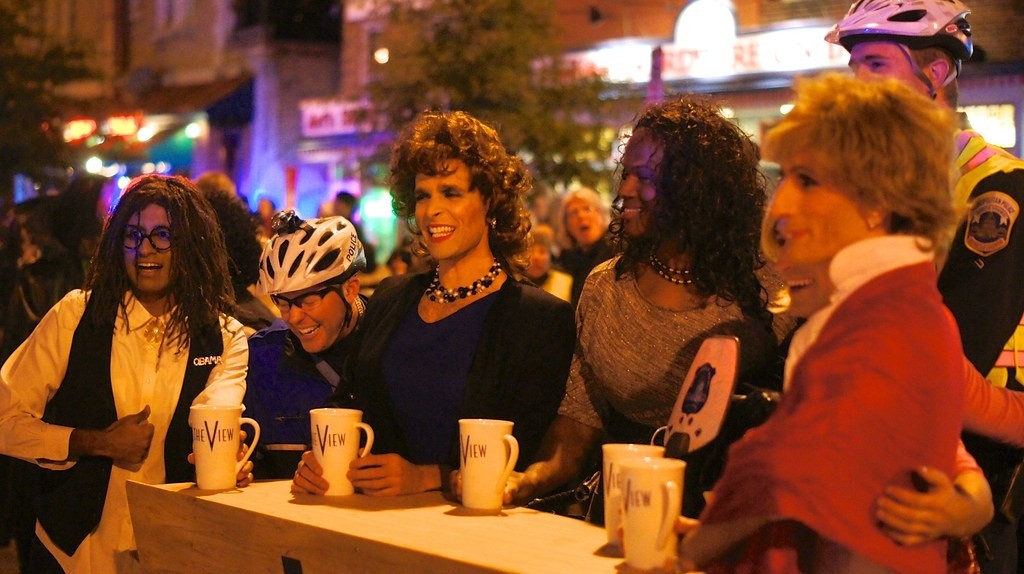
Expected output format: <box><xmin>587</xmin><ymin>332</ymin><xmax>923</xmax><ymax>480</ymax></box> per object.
<box><xmin>0</xmin><ymin>171</ymin><xmax>613</xmax><ymax>364</ymax></box>
<box><xmin>676</xmin><ymin>0</ymin><xmax>1024</xmax><ymax>574</ymax></box>
<box><xmin>242</xmin><ymin>211</ymin><xmax>369</xmax><ymax>484</ymax></box>
<box><xmin>0</xmin><ymin>173</ymin><xmax>250</xmax><ymax>574</ymax></box>
<box><xmin>289</xmin><ymin>109</ymin><xmax>574</xmax><ymax>497</ymax></box>
<box><xmin>448</xmin><ymin>95</ymin><xmax>777</xmax><ymax>535</ymax></box>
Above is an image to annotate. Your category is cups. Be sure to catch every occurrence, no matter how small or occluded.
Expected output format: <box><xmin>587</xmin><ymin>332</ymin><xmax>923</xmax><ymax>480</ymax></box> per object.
<box><xmin>459</xmin><ymin>419</ymin><xmax>518</xmax><ymax>510</ymax></box>
<box><xmin>603</xmin><ymin>443</ymin><xmax>665</xmax><ymax>546</ymax></box>
<box><xmin>310</xmin><ymin>408</ymin><xmax>374</xmax><ymax>497</ymax></box>
<box><xmin>190</xmin><ymin>404</ymin><xmax>260</xmax><ymax>491</ymax></box>
<box><xmin>619</xmin><ymin>457</ymin><xmax>686</xmax><ymax>570</ymax></box>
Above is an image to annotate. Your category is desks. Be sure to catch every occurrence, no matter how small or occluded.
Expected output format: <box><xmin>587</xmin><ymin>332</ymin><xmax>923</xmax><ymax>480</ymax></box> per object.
<box><xmin>124</xmin><ymin>479</ymin><xmax>670</xmax><ymax>574</ymax></box>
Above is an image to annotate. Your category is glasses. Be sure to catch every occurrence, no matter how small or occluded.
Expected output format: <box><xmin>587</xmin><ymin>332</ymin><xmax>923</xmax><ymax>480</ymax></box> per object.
<box><xmin>121</xmin><ymin>224</ymin><xmax>173</xmax><ymax>253</ymax></box>
<box><xmin>270</xmin><ymin>286</ymin><xmax>336</xmax><ymax>313</ymax></box>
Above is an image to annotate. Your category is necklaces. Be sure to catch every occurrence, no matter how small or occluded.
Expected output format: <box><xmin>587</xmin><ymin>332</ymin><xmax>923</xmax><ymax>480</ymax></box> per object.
<box><xmin>422</xmin><ymin>256</ymin><xmax>503</xmax><ymax>303</ymax></box>
<box><xmin>640</xmin><ymin>243</ymin><xmax>730</xmax><ymax>284</ymax></box>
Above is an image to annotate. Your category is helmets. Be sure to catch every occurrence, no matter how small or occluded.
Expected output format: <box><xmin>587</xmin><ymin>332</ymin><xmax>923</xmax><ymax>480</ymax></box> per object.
<box><xmin>257</xmin><ymin>216</ymin><xmax>366</xmax><ymax>296</ymax></box>
<box><xmin>824</xmin><ymin>0</ymin><xmax>976</xmax><ymax>62</ymax></box>
